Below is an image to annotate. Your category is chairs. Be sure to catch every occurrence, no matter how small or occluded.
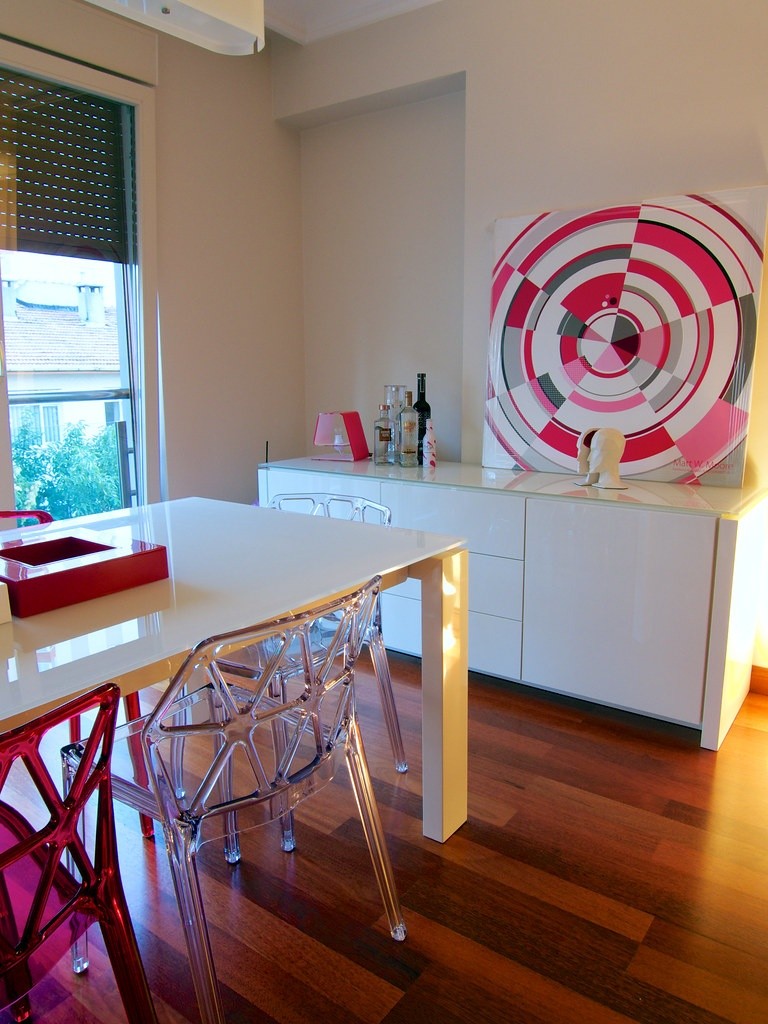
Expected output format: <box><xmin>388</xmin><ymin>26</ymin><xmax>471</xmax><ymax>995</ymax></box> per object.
<box><xmin>0</xmin><ymin>680</ymin><xmax>160</xmax><ymax>1024</ymax></box>
<box><xmin>173</xmin><ymin>493</ymin><xmax>402</xmax><ymax>852</ymax></box>
<box><xmin>0</xmin><ymin>509</ymin><xmax>153</xmax><ymax>840</ymax></box>
<box><xmin>58</xmin><ymin>575</ymin><xmax>408</xmax><ymax>1023</ymax></box>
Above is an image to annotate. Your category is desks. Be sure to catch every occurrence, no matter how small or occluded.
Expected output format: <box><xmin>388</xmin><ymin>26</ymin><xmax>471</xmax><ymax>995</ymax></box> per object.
<box><xmin>0</xmin><ymin>496</ymin><xmax>466</xmax><ymax>843</ymax></box>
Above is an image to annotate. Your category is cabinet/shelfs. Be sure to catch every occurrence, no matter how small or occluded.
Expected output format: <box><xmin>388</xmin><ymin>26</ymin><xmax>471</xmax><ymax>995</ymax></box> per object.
<box><xmin>258</xmin><ymin>451</ymin><xmax>764</xmax><ymax>752</ymax></box>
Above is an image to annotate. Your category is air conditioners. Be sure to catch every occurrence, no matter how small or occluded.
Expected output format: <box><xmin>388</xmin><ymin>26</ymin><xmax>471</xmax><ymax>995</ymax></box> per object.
<box><xmin>83</xmin><ymin>0</ymin><xmax>265</xmax><ymax>56</ymax></box>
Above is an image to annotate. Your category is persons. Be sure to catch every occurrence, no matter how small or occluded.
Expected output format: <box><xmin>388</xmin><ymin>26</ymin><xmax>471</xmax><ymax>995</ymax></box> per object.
<box><xmin>574</xmin><ymin>427</ymin><xmax>628</xmax><ymax>489</ymax></box>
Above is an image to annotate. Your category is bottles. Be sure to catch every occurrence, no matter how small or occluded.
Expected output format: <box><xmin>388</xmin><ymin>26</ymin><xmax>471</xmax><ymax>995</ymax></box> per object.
<box><xmin>422</xmin><ymin>419</ymin><xmax>436</xmax><ymax>468</ymax></box>
<box><xmin>373</xmin><ymin>404</ymin><xmax>396</xmax><ymax>465</ymax></box>
<box><xmin>413</xmin><ymin>372</ymin><xmax>432</xmax><ymax>465</ymax></box>
<box><xmin>398</xmin><ymin>391</ymin><xmax>419</xmax><ymax>467</ymax></box>
